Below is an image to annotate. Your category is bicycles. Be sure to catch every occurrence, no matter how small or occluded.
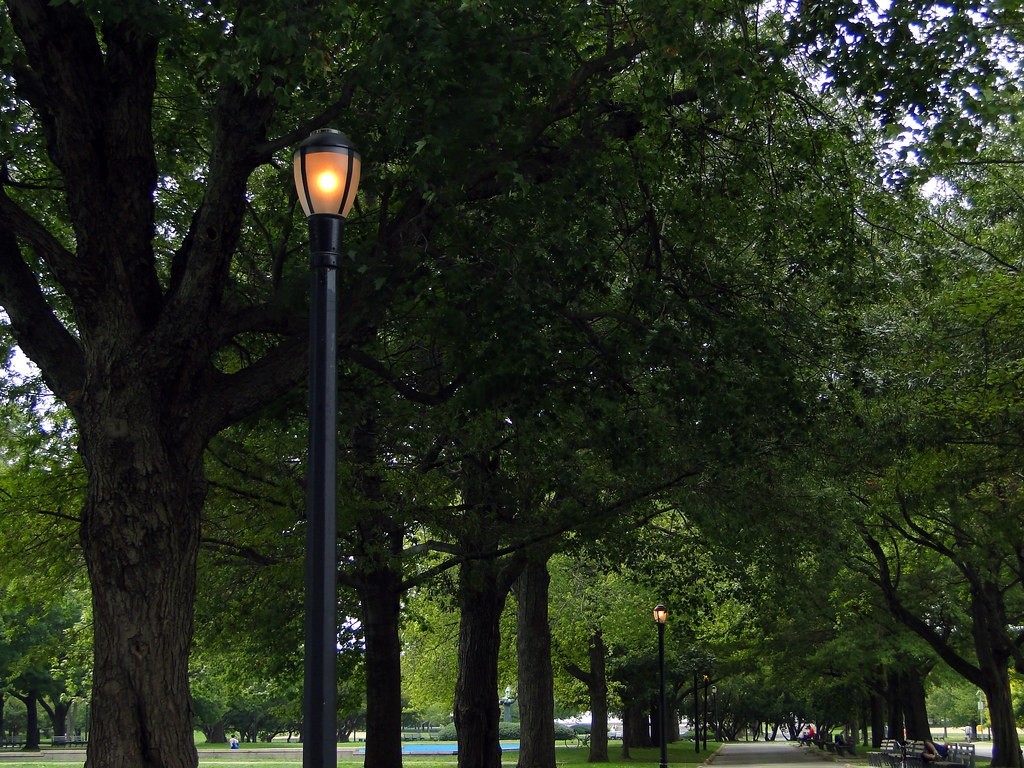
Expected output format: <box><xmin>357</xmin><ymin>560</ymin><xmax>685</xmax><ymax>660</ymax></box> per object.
<box><xmin>565</xmin><ymin>728</ymin><xmax>593</xmax><ymax>750</ymax></box>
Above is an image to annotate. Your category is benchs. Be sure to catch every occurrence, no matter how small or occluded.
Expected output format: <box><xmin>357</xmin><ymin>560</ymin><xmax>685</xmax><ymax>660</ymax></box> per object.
<box><xmin>51</xmin><ymin>736</ymin><xmax>88</xmax><ymax>748</ymax></box>
<box><xmin>5</xmin><ymin>734</ymin><xmax>26</xmax><ymax>748</ymax></box>
<box><xmin>797</xmin><ymin>732</ymin><xmax>856</xmax><ymax>757</ymax></box>
<box><xmin>866</xmin><ymin>740</ymin><xmax>975</xmax><ymax>768</ymax></box>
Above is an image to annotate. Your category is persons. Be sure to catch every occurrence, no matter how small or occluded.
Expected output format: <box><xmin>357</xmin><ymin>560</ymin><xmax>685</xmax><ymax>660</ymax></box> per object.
<box><xmin>230</xmin><ymin>735</ymin><xmax>238</xmax><ymax>749</ymax></box>
<box><xmin>798</xmin><ymin>725</ymin><xmax>814</xmax><ymax>747</ymax></box>
<box><xmin>965</xmin><ymin>724</ymin><xmax>972</xmax><ymax>743</ymax></box>
<box><xmin>920</xmin><ymin>740</ymin><xmax>948</xmax><ymax>761</ymax></box>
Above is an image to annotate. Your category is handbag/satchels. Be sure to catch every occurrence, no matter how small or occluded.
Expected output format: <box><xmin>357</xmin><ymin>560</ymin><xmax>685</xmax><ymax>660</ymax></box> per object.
<box><xmin>231</xmin><ymin>742</ymin><xmax>238</xmax><ymax>748</ymax></box>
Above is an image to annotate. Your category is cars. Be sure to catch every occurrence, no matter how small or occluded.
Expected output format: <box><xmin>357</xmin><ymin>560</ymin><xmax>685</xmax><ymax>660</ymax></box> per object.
<box><xmin>608</xmin><ymin>725</ymin><xmax>623</xmax><ymax>741</ymax></box>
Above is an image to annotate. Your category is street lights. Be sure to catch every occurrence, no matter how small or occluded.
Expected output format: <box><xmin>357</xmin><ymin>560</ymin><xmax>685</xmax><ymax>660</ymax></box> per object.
<box><xmin>652</xmin><ymin>603</ymin><xmax>670</xmax><ymax>768</ymax></box>
<box><xmin>975</xmin><ymin>690</ymin><xmax>984</xmax><ymax>742</ymax></box>
<box><xmin>291</xmin><ymin>126</ymin><xmax>364</xmax><ymax>767</ymax></box>
<box><xmin>711</xmin><ymin>685</ymin><xmax>718</xmax><ymax>743</ymax></box>
<box><xmin>703</xmin><ymin>674</ymin><xmax>709</xmax><ymax>751</ymax></box>
